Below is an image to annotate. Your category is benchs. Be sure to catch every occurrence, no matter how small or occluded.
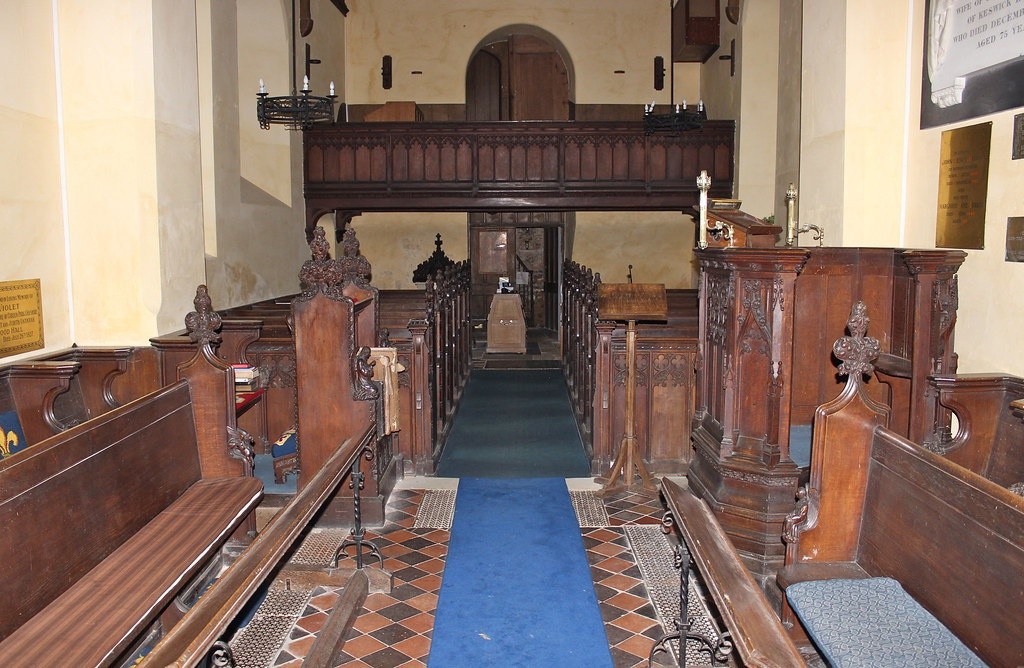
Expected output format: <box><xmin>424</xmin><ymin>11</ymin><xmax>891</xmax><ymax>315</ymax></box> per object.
<box><xmin>560</xmin><ymin>257</ymin><xmax>1024</xmax><ymax>665</ymax></box>
<box><xmin>0</xmin><ymin>257</ymin><xmax>475</xmax><ymax>668</ymax></box>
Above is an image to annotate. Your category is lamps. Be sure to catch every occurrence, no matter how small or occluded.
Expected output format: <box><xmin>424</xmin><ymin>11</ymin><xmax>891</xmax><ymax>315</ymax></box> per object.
<box><xmin>653</xmin><ymin>56</ymin><xmax>666</xmax><ymax>91</ymax></box>
<box><xmin>382</xmin><ymin>53</ymin><xmax>392</xmax><ymax>91</ymax></box>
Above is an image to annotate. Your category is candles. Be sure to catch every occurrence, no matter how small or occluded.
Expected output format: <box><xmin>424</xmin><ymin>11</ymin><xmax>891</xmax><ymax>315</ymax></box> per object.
<box><xmin>644</xmin><ymin>102</ymin><xmax>650</xmax><ymax>114</ymax></box>
<box><xmin>304</xmin><ymin>75</ymin><xmax>308</xmax><ymax>90</ymax></box>
<box><xmin>257</xmin><ymin>79</ymin><xmax>266</xmax><ymax>94</ymax></box>
<box><xmin>650</xmin><ymin>101</ymin><xmax>655</xmax><ymax>112</ymax></box>
<box><xmin>697</xmin><ymin>104</ymin><xmax>699</xmax><ymax>111</ymax></box>
<box><xmin>700</xmin><ymin>99</ymin><xmax>704</xmax><ymax>112</ymax></box>
<box><xmin>682</xmin><ymin>99</ymin><xmax>688</xmax><ymax>109</ymax></box>
<box><xmin>675</xmin><ymin>104</ymin><xmax>680</xmax><ymax>113</ymax></box>
<box><xmin>329</xmin><ymin>82</ymin><xmax>336</xmax><ymax>96</ymax></box>
